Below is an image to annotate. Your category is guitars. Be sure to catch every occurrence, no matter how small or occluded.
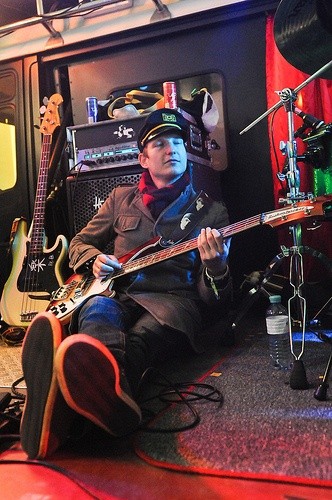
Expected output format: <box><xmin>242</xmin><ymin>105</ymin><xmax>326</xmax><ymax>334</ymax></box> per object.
<box><xmin>45</xmin><ymin>193</ymin><xmax>332</xmax><ymax>327</ymax></box>
<box><xmin>0</xmin><ymin>92</ymin><xmax>70</xmax><ymax>327</ymax></box>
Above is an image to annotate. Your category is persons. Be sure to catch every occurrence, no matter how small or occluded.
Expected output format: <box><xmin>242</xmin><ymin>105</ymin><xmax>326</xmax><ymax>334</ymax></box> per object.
<box><xmin>20</xmin><ymin>109</ymin><xmax>233</xmax><ymax>457</ymax></box>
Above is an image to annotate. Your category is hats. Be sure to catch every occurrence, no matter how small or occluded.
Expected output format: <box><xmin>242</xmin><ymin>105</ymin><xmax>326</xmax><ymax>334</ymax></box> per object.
<box><xmin>137</xmin><ymin>109</ymin><xmax>191</xmax><ymax>153</ymax></box>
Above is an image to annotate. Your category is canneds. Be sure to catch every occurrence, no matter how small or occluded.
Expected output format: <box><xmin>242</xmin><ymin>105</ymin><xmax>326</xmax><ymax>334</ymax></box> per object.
<box><xmin>163</xmin><ymin>81</ymin><xmax>177</xmax><ymax>109</ymax></box>
<box><xmin>85</xmin><ymin>96</ymin><xmax>98</xmax><ymax>124</ymax></box>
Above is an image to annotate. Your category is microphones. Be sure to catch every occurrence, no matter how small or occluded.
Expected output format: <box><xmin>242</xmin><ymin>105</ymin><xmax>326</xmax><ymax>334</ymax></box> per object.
<box><xmin>292</xmin><ymin>107</ymin><xmax>325</xmax><ymax>129</ymax></box>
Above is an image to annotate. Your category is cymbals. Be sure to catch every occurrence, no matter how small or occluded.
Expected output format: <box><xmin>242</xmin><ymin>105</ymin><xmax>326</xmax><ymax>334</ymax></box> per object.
<box><xmin>273</xmin><ymin>1</ymin><xmax>332</xmax><ymax>80</ymax></box>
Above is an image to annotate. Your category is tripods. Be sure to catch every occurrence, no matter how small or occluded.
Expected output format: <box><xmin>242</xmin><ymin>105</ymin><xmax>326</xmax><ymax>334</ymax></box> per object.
<box><xmin>221</xmin><ymin>60</ymin><xmax>332</xmax><ymax>390</ymax></box>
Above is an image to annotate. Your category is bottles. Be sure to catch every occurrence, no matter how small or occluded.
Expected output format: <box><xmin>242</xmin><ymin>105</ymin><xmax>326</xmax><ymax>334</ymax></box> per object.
<box><xmin>265</xmin><ymin>295</ymin><xmax>291</xmax><ymax>370</ymax></box>
<box><xmin>312</xmin><ymin>167</ymin><xmax>332</xmax><ymax>220</ymax></box>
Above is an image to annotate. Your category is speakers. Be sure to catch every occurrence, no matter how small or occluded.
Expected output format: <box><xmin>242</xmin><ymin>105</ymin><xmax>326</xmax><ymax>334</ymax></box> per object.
<box><xmin>65</xmin><ymin>162</ymin><xmax>219</xmax><ymax>257</ymax></box>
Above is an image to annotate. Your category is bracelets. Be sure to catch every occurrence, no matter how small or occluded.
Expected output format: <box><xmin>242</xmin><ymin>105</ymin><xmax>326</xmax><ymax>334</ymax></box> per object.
<box><xmin>205</xmin><ymin>264</ymin><xmax>230</xmax><ymax>299</ymax></box>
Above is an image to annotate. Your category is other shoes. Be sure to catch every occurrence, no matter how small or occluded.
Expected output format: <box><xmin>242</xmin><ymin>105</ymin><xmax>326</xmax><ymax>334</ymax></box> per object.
<box><xmin>18</xmin><ymin>311</ymin><xmax>66</xmax><ymax>461</ymax></box>
<box><xmin>54</xmin><ymin>332</ymin><xmax>143</xmax><ymax>439</ymax></box>
